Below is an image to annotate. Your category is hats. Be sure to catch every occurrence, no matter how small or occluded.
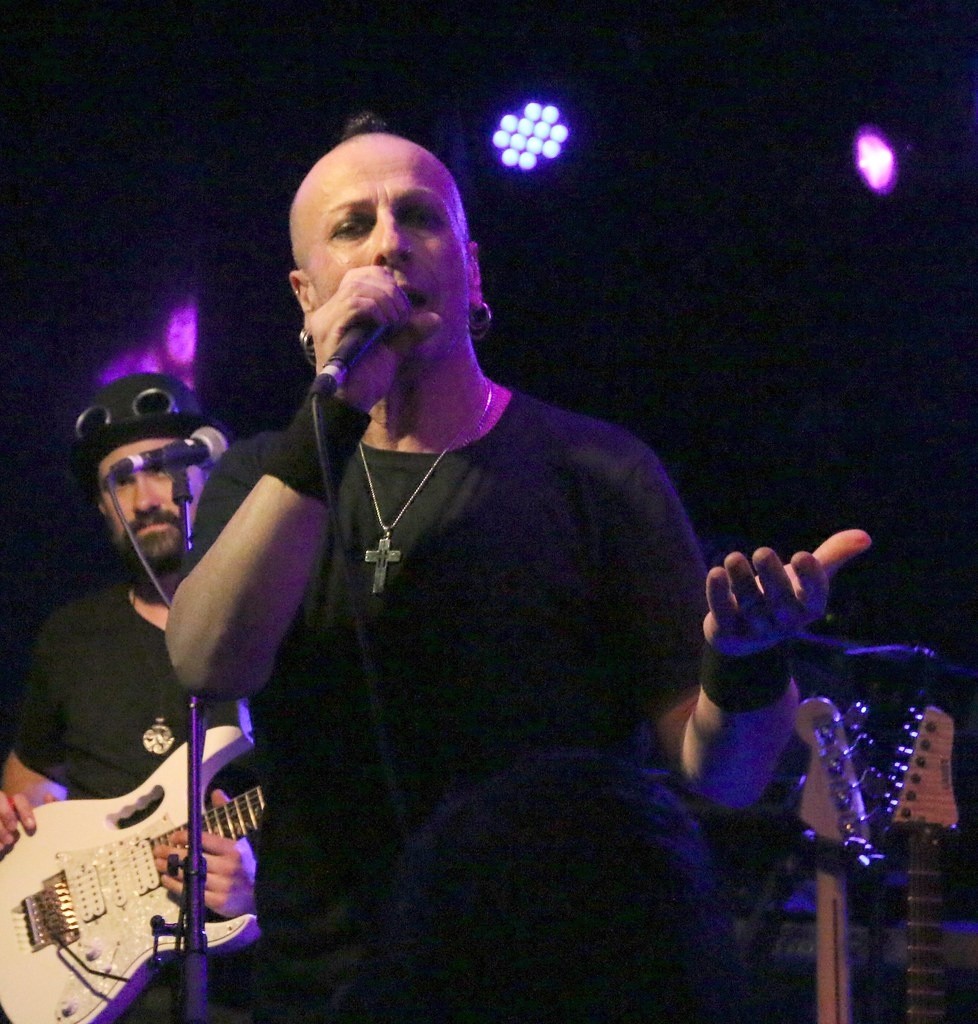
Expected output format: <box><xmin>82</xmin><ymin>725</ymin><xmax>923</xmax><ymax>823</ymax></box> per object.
<box><xmin>67</xmin><ymin>373</ymin><xmax>235</xmax><ymax>480</ymax></box>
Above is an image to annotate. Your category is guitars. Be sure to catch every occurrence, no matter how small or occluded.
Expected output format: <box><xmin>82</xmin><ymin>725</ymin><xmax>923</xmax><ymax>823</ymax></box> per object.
<box><xmin>790</xmin><ymin>693</ymin><xmax>958</xmax><ymax>1024</ymax></box>
<box><xmin>0</xmin><ymin>720</ymin><xmax>264</xmax><ymax>1024</ymax></box>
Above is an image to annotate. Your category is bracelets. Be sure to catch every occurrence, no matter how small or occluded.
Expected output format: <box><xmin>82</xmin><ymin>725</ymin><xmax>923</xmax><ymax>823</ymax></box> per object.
<box><xmin>262</xmin><ymin>390</ymin><xmax>372</xmax><ymax>508</ymax></box>
<box><xmin>699</xmin><ymin>646</ymin><xmax>792</xmax><ymax>713</ymax></box>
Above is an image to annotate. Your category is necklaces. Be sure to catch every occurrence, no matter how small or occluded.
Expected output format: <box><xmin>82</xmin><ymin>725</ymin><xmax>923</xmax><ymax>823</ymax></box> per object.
<box><xmin>358</xmin><ymin>378</ymin><xmax>490</xmax><ymax>595</ymax></box>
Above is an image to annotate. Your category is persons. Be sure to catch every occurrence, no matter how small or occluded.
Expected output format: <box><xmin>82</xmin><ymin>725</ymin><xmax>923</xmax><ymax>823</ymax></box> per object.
<box><xmin>1</xmin><ymin>373</ymin><xmax>260</xmax><ymax>1024</ymax></box>
<box><xmin>166</xmin><ymin>117</ymin><xmax>871</xmax><ymax>1023</ymax></box>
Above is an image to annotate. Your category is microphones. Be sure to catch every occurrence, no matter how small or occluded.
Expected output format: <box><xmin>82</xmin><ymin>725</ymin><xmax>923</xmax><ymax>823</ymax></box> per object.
<box><xmin>307</xmin><ymin>315</ymin><xmax>400</xmax><ymax>401</ymax></box>
<box><xmin>109</xmin><ymin>424</ymin><xmax>229</xmax><ymax>479</ymax></box>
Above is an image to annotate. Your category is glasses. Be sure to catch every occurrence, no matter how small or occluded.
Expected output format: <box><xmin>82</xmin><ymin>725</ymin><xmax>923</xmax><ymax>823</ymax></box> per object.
<box><xmin>75</xmin><ymin>387</ymin><xmax>175</xmax><ymax>436</ymax></box>
